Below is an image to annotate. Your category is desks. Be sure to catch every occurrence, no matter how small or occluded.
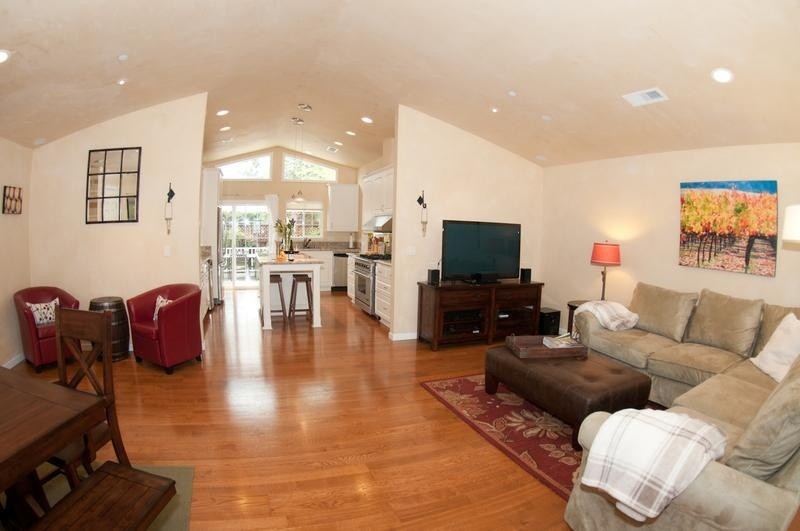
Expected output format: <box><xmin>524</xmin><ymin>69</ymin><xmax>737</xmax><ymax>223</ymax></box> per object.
<box><xmin>0</xmin><ymin>365</ymin><xmax>106</xmax><ymax>494</ymax></box>
<box><xmin>256</xmin><ymin>254</ymin><xmax>324</xmax><ymax>329</ymax></box>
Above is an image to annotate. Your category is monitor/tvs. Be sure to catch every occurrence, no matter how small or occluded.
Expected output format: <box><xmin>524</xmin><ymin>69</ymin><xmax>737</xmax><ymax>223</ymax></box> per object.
<box><xmin>440</xmin><ymin>220</ymin><xmax>521</xmax><ymax>285</ymax></box>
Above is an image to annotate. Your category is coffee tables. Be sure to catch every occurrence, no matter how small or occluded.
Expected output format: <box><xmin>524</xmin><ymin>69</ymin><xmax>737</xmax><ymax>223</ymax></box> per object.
<box><xmin>485</xmin><ymin>345</ymin><xmax>652</xmax><ymax>450</ymax></box>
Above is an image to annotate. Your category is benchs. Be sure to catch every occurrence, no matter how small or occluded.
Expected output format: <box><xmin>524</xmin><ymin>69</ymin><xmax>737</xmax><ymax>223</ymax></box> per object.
<box><xmin>28</xmin><ymin>460</ymin><xmax>177</xmax><ymax>531</ymax></box>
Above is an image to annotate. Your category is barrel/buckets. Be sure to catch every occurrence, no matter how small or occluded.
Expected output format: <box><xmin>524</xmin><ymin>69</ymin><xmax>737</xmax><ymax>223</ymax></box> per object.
<box><xmin>89</xmin><ymin>297</ymin><xmax>130</xmax><ymax>362</ymax></box>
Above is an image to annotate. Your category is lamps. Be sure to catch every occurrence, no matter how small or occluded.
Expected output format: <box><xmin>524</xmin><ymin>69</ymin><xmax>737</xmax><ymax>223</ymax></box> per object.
<box><xmin>291</xmin><ymin>118</ymin><xmax>304</xmax><ymax>202</ymax></box>
<box><xmin>592</xmin><ymin>242</ymin><xmax>621</xmax><ymax>300</ymax></box>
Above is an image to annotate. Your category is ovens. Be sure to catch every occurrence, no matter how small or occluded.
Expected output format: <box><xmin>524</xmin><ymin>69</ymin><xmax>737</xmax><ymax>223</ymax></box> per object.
<box><xmin>352</xmin><ymin>259</ymin><xmax>375</xmax><ymax>315</ymax></box>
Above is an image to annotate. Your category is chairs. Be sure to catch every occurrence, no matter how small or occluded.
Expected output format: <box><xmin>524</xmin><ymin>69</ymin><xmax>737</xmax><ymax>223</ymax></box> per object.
<box><xmin>44</xmin><ymin>305</ymin><xmax>132</xmax><ymax>489</ymax></box>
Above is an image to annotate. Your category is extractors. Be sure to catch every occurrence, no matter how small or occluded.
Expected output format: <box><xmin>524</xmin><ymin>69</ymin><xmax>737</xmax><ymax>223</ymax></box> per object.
<box><xmin>361</xmin><ymin>216</ymin><xmax>392</xmax><ymax>233</ymax></box>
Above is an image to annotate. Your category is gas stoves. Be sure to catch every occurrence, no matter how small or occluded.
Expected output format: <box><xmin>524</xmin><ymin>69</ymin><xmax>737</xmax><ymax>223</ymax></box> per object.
<box><xmin>355</xmin><ymin>253</ymin><xmax>391</xmax><ymax>263</ymax></box>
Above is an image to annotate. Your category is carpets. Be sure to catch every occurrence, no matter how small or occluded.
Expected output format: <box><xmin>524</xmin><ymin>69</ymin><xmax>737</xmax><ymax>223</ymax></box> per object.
<box><xmin>420</xmin><ymin>374</ymin><xmax>664</xmax><ymax>501</ymax></box>
<box><xmin>44</xmin><ymin>462</ymin><xmax>194</xmax><ymax>531</ymax></box>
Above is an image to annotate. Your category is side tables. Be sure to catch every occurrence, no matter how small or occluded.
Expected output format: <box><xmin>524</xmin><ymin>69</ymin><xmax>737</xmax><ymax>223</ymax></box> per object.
<box><xmin>567</xmin><ymin>300</ymin><xmax>590</xmax><ymax>335</ymax></box>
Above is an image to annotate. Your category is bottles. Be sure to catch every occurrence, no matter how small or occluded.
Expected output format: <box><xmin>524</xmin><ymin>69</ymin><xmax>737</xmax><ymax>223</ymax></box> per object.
<box><xmin>288</xmin><ymin>240</ymin><xmax>294</xmax><ymax>261</ymax></box>
<box><xmin>280</xmin><ymin>242</ymin><xmax>285</xmax><ymax>257</ymax></box>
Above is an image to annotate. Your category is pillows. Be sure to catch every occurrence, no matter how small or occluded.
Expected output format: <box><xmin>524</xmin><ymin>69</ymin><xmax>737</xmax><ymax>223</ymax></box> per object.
<box><xmin>628</xmin><ymin>281</ymin><xmax>698</xmax><ymax>344</ymax></box>
<box><xmin>25</xmin><ymin>296</ymin><xmax>60</xmax><ymax>324</ymax></box>
<box><xmin>725</xmin><ymin>353</ymin><xmax>800</xmax><ymax>482</ymax></box>
<box><xmin>748</xmin><ymin>310</ymin><xmax>800</xmax><ymax>382</ymax></box>
<box><xmin>683</xmin><ymin>288</ymin><xmax>763</xmax><ymax>359</ymax></box>
<box><xmin>152</xmin><ymin>295</ymin><xmax>172</xmax><ymax>320</ymax></box>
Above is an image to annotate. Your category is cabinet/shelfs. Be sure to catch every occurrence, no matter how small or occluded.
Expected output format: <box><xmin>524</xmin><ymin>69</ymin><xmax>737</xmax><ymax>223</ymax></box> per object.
<box><xmin>374</xmin><ymin>263</ymin><xmax>393</xmax><ymax>322</ymax></box>
<box><xmin>347</xmin><ymin>257</ymin><xmax>356</xmax><ymax>299</ymax></box>
<box><xmin>327</xmin><ymin>163</ymin><xmax>394</xmax><ymax>232</ymax></box>
<box><xmin>417</xmin><ymin>280</ymin><xmax>545</xmax><ymax>351</ymax></box>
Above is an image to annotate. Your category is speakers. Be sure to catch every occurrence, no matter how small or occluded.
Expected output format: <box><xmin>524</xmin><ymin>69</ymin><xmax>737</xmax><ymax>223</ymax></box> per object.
<box><xmin>428</xmin><ymin>270</ymin><xmax>439</xmax><ymax>284</ymax></box>
<box><xmin>520</xmin><ymin>268</ymin><xmax>531</xmax><ymax>284</ymax></box>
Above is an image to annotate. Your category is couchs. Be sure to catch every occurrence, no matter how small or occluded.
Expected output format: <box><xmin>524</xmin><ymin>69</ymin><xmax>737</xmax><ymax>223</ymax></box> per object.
<box><xmin>127</xmin><ymin>283</ymin><xmax>203</xmax><ymax>374</ymax></box>
<box><xmin>13</xmin><ymin>287</ymin><xmax>80</xmax><ymax>373</ymax></box>
<box><xmin>573</xmin><ymin>300</ymin><xmax>800</xmax><ymax>531</ymax></box>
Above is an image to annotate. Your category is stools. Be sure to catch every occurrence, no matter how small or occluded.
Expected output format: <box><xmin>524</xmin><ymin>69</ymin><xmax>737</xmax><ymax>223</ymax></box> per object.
<box><xmin>271</xmin><ymin>275</ymin><xmax>287</xmax><ymax>321</ymax></box>
<box><xmin>288</xmin><ymin>274</ymin><xmax>312</xmax><ymax>320</ymax></box>
